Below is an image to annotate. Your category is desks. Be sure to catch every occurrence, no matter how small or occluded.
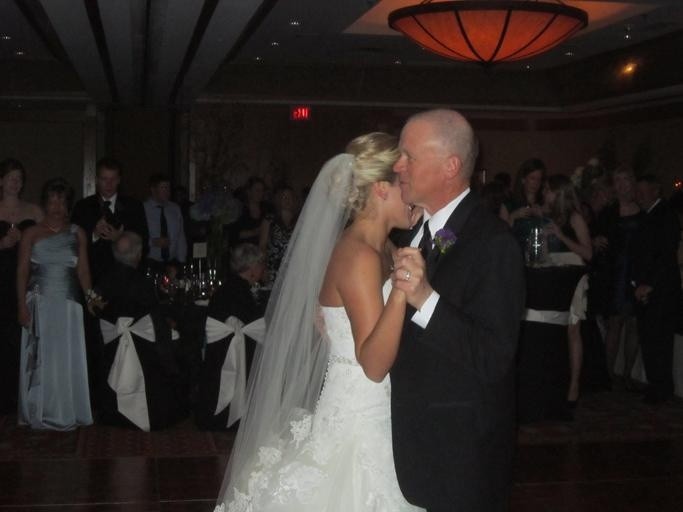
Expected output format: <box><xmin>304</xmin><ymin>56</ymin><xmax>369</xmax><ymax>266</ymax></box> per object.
<box><xmin>509</xmin><ymin>263</ymin><xmax>590</xmax><ymax>389</ymax></box>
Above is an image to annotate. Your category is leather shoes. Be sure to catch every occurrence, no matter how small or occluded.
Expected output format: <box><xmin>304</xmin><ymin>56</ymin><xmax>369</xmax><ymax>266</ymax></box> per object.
<box><xmin>565</xmin><ymin>388</ymin><xmax>580</xmax><ymax>410</ymax></box>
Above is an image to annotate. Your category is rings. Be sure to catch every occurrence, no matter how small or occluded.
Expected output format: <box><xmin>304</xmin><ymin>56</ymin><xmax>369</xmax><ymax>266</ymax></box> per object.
<box><xmin>404</xmin><ymin>272</ymin><xmax>410</xmax><ymax>281</ymax></box>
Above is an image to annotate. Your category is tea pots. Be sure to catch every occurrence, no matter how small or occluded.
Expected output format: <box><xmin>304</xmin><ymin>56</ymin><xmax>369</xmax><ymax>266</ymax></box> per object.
<box><xmin>526</xmin><ymin>222</ymin><xmax>544</xmax><ymax>265</ymax></box>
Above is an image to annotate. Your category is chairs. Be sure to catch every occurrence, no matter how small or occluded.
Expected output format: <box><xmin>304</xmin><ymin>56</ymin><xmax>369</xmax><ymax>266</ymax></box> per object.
<box><xmin>90</xmin><ymin>279</ymin><xmax>262</xmax><ymax>434</ymax></box>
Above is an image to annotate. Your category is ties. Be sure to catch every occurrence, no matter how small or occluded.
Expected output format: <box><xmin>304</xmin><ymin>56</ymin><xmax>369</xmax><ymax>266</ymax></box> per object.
<box><xmin>158</xmin><ymin>206</ymin><xmax>169</xmax><ymax>263</ymax></box>
<box><xmin>102</xmin><ymin>201</ymin><xmax>112</xmax><ymax>219</ymax></box>
<box><xmin>417</xmin><ymin>219</ymin><xmax>432</xmax><ymax>260</ymax></box>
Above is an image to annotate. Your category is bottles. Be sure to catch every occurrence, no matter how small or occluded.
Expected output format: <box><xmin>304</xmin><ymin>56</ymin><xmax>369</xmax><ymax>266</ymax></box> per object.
<box><xmin>160</xmin><ymin>261</ymin><xmax>225</xmax><ymax>303</ymax></box>
<box><xmin>629</xmin><ymin>278</ymin><xmax>650</xmax><ymax>309</ymax></box>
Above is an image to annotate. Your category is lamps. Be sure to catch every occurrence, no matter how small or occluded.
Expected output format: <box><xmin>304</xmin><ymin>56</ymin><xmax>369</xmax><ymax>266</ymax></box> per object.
<box><xmin>386</xmin><ymin>0</ymin><xmax>588</xmax><ymax>64</ymax></box>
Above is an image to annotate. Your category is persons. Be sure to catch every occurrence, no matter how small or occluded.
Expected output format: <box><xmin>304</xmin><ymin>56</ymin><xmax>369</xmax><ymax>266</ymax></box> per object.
<box><xmin>18</xmin><ymin>177</ymin><xmax>104</xmax><ymax>431</ymax></box>
<box><xmin>493</xmin><ymin>162</ymin><xmax>592</xmax><ymax>404</ymax></box>
<box><xmin>73</xmin><ymin>162</ymin><xmax>299</xmax><ymax>432</ymax></box>
<box><xmin>313</xmin><ymin>108</ymin><xmax>526</xmax><ymax>512</ymax></box>
<box><xmin>0</xmin><ymin>161</ymin><xmax>46</xmax><ymax>415</ymax></box>
<box><xmin>214</xmin><ymin>132</ymin><xmax>424</xmax><ymax>512</ymax></box>
<box><xmin>577</xmin><ymin>164</ymin><xmax>683</xmax><ymax>403</ymax></box>
